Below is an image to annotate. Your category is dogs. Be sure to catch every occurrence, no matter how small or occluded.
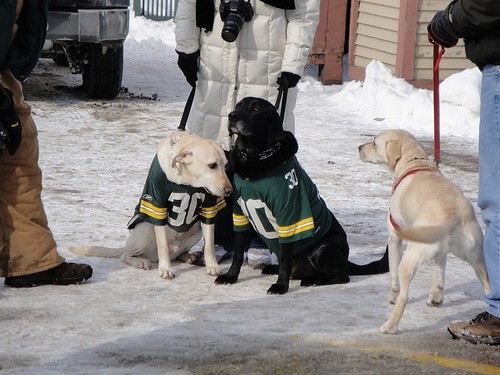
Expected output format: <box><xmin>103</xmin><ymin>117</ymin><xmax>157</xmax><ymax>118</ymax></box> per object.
<box><xmin>66</xmin><ymin>131</ymin><xmax>234</xmax><ymax>280</ymax></box>
<box><xmin>358</xmin><ymin>128</ymin><xmax>492</xmax><ymax>335</ymax></box>
<box><xmin>213</xmin><ymin>96</ymin><xmax>389</xmax><ymax>295</ymax></box>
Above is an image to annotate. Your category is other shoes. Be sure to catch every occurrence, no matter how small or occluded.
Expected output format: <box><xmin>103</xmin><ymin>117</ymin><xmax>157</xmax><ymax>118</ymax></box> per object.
<box><xmin>198</xmin><ymin>244</ymin><xmax>227</xmax><ymax>266</ymax></box>
<box><xmin>243</xmin><ymin>246</ymin><xmax>272</xmax><ymax>269</ymax></box>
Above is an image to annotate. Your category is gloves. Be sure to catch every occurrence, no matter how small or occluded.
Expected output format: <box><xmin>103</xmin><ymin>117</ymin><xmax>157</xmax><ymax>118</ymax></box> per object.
<box><xmin>175</xmin><ymin>49</ymin><xmax>200</xmax><ymax>88</ymax></box>
<box><xmin>0</xmin><ymin>84</ymin><xmax>22</xmax><ymax>156</ymax></box>
<box><xmin>427</xmin><ymin>0</ymin><xmax>459</xmax><ymax>48</ymax></box>
<box><xmin>276</xmin><ymin>71</ymin><xmax>301</xmax><ymax>91</ymax></box>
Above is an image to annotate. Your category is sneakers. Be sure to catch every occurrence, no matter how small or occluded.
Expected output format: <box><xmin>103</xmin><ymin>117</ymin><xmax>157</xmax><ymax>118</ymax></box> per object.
<box><xmin>447</xmin><ymin>311</ymin><xmax>500</xmax><ymax>344</ymax></box>
<box><xmin>4</xmin><ymin>261</ymin><xmax>93</xmax><ymax>289</ymax></box>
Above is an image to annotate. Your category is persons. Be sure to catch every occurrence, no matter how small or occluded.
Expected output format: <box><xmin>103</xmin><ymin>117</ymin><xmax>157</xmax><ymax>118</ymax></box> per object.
<box><xmin>173</xmin><ymin>0</ymin><xmax>320</xmax><ymax>269</ymax></box>
<box><xmin>0</xmin><ymin>0</ymin><xmax>93</xmax><ymax>288</ymax></box>
<box><xmin>427</xmin><ymin>0</ymin><xmax>500</xmax><ymax>346</ymax></box>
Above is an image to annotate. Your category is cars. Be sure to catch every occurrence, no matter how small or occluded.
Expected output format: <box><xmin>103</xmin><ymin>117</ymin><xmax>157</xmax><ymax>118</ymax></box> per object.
<box><xmin>44</xmin><ymin>0</ymin><xmax>131</xmax><ymax>100</ymax></box>
